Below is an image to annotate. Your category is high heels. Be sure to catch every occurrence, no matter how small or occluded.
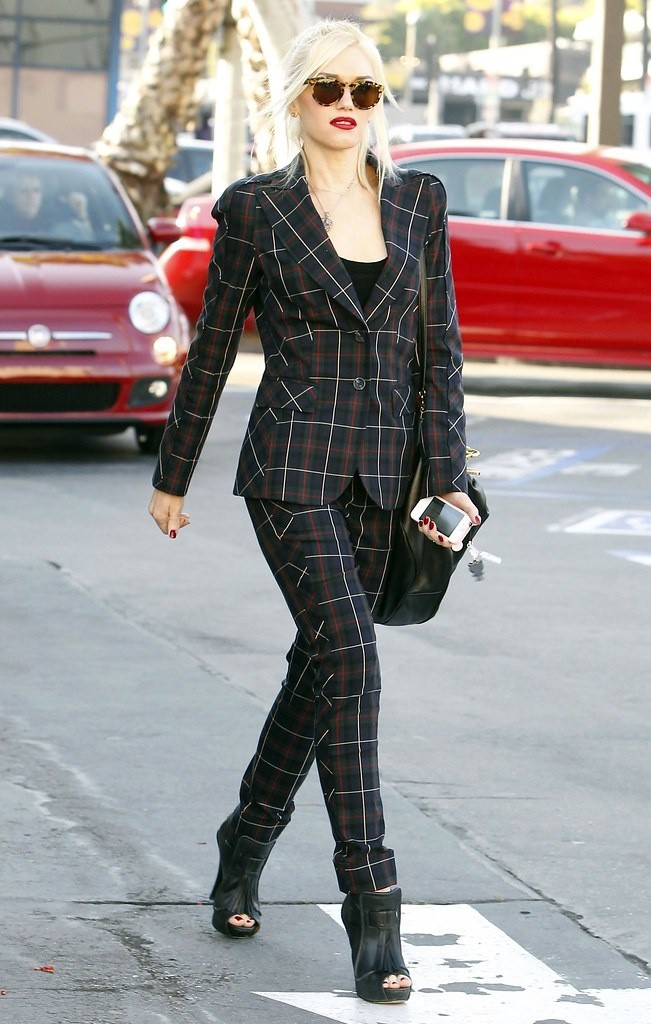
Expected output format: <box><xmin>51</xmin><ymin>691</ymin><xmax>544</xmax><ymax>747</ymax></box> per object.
<box><xmin>341</xmin><ymin>887</ymin><xmax>413</xmax><ymax>1005</ymax></box>
<box><xmin>209</xmin><ymin>802</ymin><xmax>276</xmax><ymax>939</ymax></box>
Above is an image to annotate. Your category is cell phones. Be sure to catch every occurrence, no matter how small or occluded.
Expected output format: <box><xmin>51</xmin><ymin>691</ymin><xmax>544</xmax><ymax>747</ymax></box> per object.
<box><xmin>410</xmin><ymin>495</ymin><xmax>473</xmax><ymax>544</ymax></box>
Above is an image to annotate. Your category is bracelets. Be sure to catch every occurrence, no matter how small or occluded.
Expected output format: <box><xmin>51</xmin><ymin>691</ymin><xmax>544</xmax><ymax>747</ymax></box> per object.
<box><xmin>79</xmin><ymin>218</ymin><xmax>89</xmax><ymax>223</ymax></box>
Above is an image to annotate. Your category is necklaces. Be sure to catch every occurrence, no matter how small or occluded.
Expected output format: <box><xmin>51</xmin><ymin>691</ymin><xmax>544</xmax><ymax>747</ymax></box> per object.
<box><xmin>305</xmin><ymin>176</ymin><xmax>358</xmax><ymax>232</ymax></box>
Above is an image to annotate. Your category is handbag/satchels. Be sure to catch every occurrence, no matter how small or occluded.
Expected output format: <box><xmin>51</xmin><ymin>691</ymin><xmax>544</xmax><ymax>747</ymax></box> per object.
<box><xmin>372</xmin><ymin>455</ymin><xmax>492</xmax><ymax>626</ymax></box>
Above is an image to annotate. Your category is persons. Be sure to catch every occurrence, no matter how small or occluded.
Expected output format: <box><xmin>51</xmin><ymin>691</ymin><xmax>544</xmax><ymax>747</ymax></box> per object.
<box><xmin>146</xmin><ymin>17</ymin><xmax>487</xmax><ymax>1006</ymax></box>
<box><xmin>0</xmin><ymin>169</ymin><xmax>96</xmax><ymax>246</ymax></box>
<box><xmin>531</xmin><ymin>175</ymin><xmax>621</xmax><ymax>230</ymax></box>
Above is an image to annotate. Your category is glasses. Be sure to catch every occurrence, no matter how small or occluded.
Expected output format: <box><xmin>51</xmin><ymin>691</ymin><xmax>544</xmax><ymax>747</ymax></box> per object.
<box><xmin>303</xmin><ymin>77</ymin><xmax>385</xmax><ymax>111</ymax></box>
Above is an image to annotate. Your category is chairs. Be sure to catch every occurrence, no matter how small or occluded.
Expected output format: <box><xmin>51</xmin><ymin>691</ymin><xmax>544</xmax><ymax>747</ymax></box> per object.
<box><xmin>537</xmin><ymin>177</ymin><xmax>573</xmax><ymax>224</ymax></box>
